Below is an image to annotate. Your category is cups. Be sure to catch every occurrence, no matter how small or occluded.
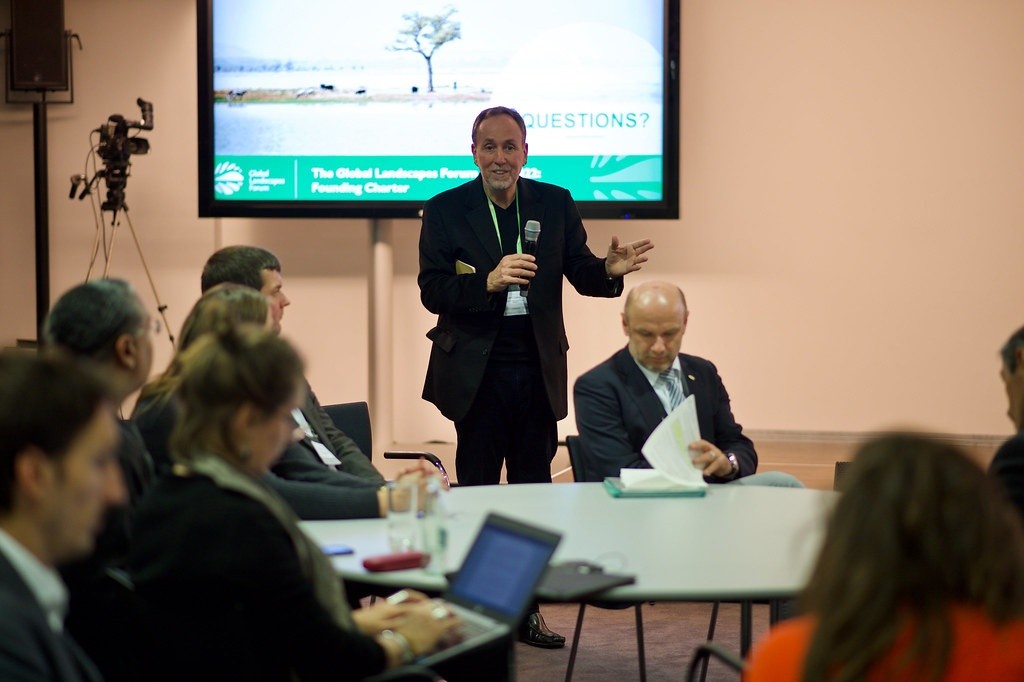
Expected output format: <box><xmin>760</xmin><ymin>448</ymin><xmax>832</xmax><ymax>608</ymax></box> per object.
<box><xmin>384</xmin><ymin>482</ymin><xmax>436</xmax><ymax>553</ymax></box>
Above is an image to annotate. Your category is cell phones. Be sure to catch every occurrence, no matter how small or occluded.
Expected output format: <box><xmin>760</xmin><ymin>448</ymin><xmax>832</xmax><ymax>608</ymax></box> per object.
<box><xmin>322</xmin><ymin>544</ymin><xmax>353</xmax><ymax>554</ymax></box>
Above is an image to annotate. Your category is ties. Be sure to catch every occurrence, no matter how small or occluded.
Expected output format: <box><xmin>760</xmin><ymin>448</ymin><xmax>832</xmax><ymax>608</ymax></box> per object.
<box><xmin>660</xmin><ymin>367</ymin><xmax>685</xmax><ymax>411</ymax></box>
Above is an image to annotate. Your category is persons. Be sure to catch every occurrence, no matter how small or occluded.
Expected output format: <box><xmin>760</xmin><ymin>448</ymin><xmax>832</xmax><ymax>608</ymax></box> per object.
<box><xmin>46</xmin><ymin>277</ymin><xmax>159</xmax><ymax>499</ymax></box>
<box><xmin>988</xmin><ymin>328</ymin><xmax>1024</xmax><ymax>525</ymax></box>
<box><xmin>574</xmin><ymin>282</ymin><xmax>811</xmax><ymax>629</ymax></box>
<box><xmin>0</xmin><ymin>339</ymin><xmax>130</xmax><ymax>682</ymax></box>
<box><xmin>132</xmin><ymin>246</ymin><xmax>439</xmax><ymax>518</ymax></box>
<box><xmin>138</xmin><ymin>324</ymin><xmax>466</xmax><ymax>682</ymax></box>
<box><xmin>416</xmin><ymin>107</ymin><xmax>654</xmax><ymax>648</ymax></box>
<box><xmin>743</xmin><ymin>431</ymin><xmax>1023</xmax><ymax>682</ymax></box>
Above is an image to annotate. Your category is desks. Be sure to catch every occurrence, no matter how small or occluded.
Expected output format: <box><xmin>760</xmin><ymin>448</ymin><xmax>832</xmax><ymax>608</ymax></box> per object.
<box><xmin>293</xmin><ymin>483</ymin><xmax>845</xmax><ymax>606</ymax></box>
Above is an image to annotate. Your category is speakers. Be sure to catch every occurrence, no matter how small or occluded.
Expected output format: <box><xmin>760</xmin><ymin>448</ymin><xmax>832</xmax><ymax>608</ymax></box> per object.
<box><xmin>10</xmin><ymin>0</ymin><xmax>69</xmax><ymax>91</ymax></box>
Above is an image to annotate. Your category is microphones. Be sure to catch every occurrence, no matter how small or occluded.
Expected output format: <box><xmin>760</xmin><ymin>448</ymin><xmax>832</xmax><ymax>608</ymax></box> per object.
<box><xmin>519</xmin><ymin>221</ymin><xmax>541</xmax><ymax>297</ymax></box>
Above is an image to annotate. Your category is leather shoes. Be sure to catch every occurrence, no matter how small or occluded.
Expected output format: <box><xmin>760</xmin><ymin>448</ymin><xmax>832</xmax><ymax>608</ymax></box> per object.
<box><xmin>519</xmin><ymin>612</ymin><xmax>566</xmax><ymax>648</ymax></box>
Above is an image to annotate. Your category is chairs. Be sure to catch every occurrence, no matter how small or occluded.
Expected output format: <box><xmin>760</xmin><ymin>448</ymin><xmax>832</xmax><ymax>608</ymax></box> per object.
<box><xmin>567</xmin><ymin>433</ymin><xmax>721</xmax><ymax>682</ymax></box>
<box><xmin>302</xmin><ymin>401</ymin><xmax>448</xmax><ymax>486</ymax></box>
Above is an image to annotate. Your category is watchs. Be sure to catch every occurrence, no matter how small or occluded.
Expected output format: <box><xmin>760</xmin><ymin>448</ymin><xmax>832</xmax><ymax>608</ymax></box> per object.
<box><xmin>728</xmin><ymin>452</ymin><xmax>739</xmax><ymax>471</ymax></box>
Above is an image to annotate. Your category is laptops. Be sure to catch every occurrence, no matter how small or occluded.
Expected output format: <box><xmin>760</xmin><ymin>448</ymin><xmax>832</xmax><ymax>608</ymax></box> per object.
<box><xmin>410</xmin><ymin>511</ymin><xmax>562</xmax><ymax>668</ymax></box>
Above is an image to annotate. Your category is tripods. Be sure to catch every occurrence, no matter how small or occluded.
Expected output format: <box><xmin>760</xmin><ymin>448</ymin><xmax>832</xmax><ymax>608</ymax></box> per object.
<box><xmin>69</xmin><ymin>160</ymin><xmax>176</xmax><ymax>351</ymax></box>
